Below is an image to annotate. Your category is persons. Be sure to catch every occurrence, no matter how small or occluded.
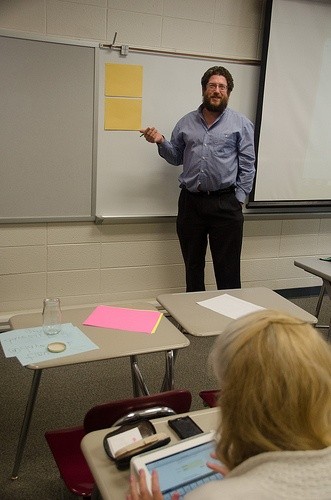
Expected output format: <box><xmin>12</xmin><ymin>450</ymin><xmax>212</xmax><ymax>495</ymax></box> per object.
<box><xmin>137</xmin><ymin>65</ymin><xmax>256</xmax><ymax>295</ymax></box>
<box><xmin>123</xmin><ymin>308</ymin><xmax>331</xmax><ymax>500</ymax></box>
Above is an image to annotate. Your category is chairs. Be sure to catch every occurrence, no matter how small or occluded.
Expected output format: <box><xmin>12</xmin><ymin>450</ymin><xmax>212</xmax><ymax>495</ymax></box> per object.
<box><xmin>44</xmin><ymin>390</ymin><xmax>192</xmax><ymax>500</ymax></box>
<box><xmin>199</xmin><ymin>390</ymin><xmax>221</xmax><ymax>408</ymax></box>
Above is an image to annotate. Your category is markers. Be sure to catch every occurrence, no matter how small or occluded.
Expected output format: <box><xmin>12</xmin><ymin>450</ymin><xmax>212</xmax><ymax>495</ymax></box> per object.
<box><xmin>139</xmin><ymin>133</ymin><xmax>144</xmax><ymax>138</ymax></box>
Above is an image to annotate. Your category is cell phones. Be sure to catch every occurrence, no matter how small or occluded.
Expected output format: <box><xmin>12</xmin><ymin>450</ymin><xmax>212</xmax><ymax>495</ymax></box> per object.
<box><xmin>168</xmin><ymin>416</ymin><xmax>204</xmax><ymax>439</ymax></box>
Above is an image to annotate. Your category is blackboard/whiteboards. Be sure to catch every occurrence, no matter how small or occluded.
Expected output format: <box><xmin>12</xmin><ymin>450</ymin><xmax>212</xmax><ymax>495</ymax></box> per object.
<box><xmin>0</xmin><ymin>32</ymin><xmax>330</xmax><ymax>230</ymax></box>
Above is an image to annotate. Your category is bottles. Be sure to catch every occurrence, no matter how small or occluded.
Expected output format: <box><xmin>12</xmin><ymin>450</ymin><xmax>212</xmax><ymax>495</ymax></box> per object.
<box><xmin>41</xmin><ymin>297</ymin><xmax>63</xmax><ymax>336</ymax></box>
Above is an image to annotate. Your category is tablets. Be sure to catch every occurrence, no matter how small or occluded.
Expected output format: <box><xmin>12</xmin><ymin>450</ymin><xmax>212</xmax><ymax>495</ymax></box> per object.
<box><xmin>132</xmin><ymin>432</ymin><xmax>227</xmax><ymax>500</ymax></box>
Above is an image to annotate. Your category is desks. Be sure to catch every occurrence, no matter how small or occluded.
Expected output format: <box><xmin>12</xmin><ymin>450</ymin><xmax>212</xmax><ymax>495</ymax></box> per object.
<box><xmin>9</xmin><ymin>302</ymin><xmax>190</xmax><ymax>480</ymax></box>
<box><xmin>159</xmin><ymin>286</ymin><xmax>318</xmax><ymax>393</ymax></box>
<box><xmin>294</xmin><ymin>258</ymin><xmax>331</xmax><ymax>342</ymax></box>
<box><xmin>80</xmin><ymin>407</ymin><xmax>224</xmax><ymax>500</ymax></box>
